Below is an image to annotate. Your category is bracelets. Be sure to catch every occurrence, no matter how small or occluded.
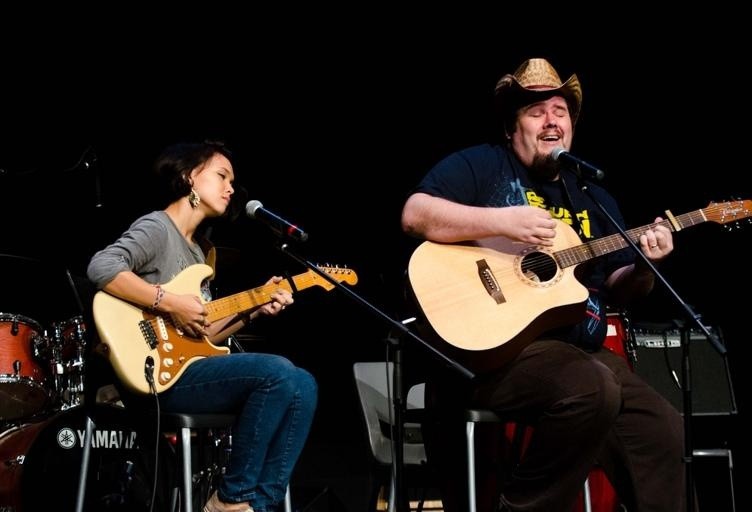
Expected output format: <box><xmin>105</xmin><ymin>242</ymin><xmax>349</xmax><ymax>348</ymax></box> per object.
<box><xmin>147</xmin><ymin>284</ymin><xmax>165</xmax><ymax>311</ymax></box>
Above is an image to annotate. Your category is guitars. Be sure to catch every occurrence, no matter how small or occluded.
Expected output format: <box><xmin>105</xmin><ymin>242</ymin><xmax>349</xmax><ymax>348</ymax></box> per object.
<box><xmin>92</xmin><ymin>263</ymin><xmax>358</xmax><ymax>395</ymax></box>
<box><xmin>406</xmin><ymin>198</ymin><xmax>752</xmax><ymax>351</ymax></box>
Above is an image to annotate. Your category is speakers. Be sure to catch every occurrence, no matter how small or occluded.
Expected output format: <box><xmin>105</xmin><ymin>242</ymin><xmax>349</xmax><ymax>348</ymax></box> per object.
<box><xmin>628</xmin><ymin>326</ymin><xmax>739</xmax><ymax>417</ymax></box>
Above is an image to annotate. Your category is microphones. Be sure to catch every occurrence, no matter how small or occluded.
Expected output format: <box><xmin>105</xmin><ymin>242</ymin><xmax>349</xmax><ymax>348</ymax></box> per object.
<box><xmin>245</xmin><ymin>199</ymin><xmax>309</xmax><ymax>242</ymax></box>
<box><xmin>551</xmin><ymin>147</ymin><xmax>604</xmax><ymax>179</ymax></box>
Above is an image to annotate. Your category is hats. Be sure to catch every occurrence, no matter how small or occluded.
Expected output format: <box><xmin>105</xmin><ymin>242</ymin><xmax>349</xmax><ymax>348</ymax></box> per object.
<box><xmin>493</xmin><ymin>55</ymin><xmax>582</xmax><ymax>116</ymax></box>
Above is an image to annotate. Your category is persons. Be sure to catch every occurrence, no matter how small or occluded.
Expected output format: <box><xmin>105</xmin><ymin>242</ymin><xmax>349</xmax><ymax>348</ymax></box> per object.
<box><xmin>86</xmin><ymin>139</ymin><xmax>316</xmax><ymax>512</ymax></box>
<box><xmin>400</xmin><ymin>50</ymin><xmax>685</xmax><ymax>512</ymax></box>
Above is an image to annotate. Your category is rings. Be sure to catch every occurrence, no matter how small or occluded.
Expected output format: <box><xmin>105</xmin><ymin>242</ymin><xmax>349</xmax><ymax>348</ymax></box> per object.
<box><xmin>649</xmin><ymin>244</ymin><xmax>658</xmax><ymax>249</ymax></box>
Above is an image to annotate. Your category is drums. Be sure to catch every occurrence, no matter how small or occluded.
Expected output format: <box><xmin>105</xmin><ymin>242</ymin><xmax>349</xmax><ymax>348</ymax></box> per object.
<box><xmin>50</xmin><ymin>315</ymin><xmax>88</xmax><ymax>373</ymax></box>
<box><xmin>0</xmin><ymin>403</ymin><xmax>183</xmax><ymax>511</ymax></box>
<box><xmin>1</xmin><ymin>312</ymin><xmax>50</xmax><ymax>421</ymax></box>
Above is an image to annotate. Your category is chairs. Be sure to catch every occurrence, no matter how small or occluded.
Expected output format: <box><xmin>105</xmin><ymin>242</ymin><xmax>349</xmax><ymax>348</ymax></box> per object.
<box><xmin>388</xmin><ymin>317</ymin><xmax>592</xmax><ymax>512</ymax></box>
<box><xmin>64</xmin><ymin>264</ymin><xmax>291</xmax><ymax>512</ymax></box>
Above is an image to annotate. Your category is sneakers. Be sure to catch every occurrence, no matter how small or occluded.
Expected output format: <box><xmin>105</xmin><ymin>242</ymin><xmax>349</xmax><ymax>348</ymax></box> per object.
<box><xmin>201</xmin><ymin>491</ymin><xmax>254</xmax><ymax>512</ymax></box>
